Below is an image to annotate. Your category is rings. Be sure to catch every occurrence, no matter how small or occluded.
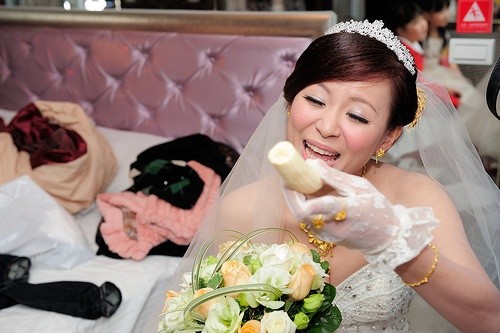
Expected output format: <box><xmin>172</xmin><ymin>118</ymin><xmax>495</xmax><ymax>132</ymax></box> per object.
<box><xmin>311</xmin><ymin>214</ymin><xmax>325</xmax><ymax>231</ymax></box>
<box><xmin>334</xmin><ymin>200</ymin><xmax>349</xmax><ymax>221</ymax></box>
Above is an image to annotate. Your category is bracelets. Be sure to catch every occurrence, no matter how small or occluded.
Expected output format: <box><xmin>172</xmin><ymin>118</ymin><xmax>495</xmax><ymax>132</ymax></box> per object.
<box><xmin>405</xmin><ymin>243</ymin><xmax>438</xmax><ymax>286</ymax></box>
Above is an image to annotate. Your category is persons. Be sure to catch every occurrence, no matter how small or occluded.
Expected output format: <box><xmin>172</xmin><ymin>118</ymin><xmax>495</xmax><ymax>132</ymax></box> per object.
<box><xmin>217</xmin><ymin>19</ymin><xmax>500</xmax><ymax>333</ymax></box>
<box><xmin>372</xmin><ymin>0</ymin><xmax>500</xmax><ymax>170</ymax></box>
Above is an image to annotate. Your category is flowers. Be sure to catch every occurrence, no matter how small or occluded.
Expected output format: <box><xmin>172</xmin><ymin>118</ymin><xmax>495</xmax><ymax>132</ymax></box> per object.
<box><xmin>158</xmin><ymin>227</ymin><xmax>342</xmax><ymax>333</ymax></box>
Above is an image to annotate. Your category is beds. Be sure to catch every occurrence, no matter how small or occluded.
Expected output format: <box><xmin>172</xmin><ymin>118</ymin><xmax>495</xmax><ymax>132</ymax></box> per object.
<box><xmin>0</xmin><ymin>5</ymin><xmax>338</xmax><ymax>333</ymax></box>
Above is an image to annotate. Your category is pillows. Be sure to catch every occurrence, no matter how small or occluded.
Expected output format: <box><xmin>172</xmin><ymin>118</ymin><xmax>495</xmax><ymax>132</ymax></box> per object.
<box><xmin>0</xmin><ymin>175</ymin><xmax>96</xmax><ymax>272</ymax></box>
<box><xmin>98</xmin><ymin>125</ymin><xmax>174</xmax><ymax>195</ymax></box>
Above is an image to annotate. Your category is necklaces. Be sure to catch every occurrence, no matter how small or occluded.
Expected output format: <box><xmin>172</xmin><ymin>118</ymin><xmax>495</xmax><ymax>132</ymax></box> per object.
<box><xmin>299</xmin><ymin>166</ymin><xmax>366</xmax><ymax>258</ymax></box>
